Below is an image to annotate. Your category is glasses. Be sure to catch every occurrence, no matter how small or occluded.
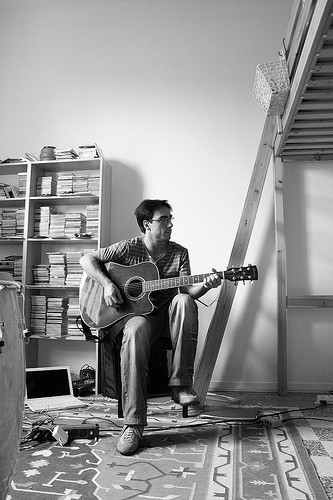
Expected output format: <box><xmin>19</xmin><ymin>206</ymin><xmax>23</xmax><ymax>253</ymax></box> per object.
<box><xmin>149</xmin><ymin>217</ymin><xmax>174</xmax><ymax>226</ymax></box>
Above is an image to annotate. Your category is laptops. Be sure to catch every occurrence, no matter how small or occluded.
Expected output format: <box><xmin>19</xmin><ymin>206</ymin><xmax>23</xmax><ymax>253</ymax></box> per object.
<box><xmin>25</xmin><ymin>366</ymin><xmax>86</xmax><ymax>412</ymax></box>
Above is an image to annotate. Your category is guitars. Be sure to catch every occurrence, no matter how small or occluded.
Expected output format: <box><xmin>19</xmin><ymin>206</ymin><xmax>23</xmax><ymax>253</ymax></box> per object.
<box><xmin>78</xmin><ymin>260</ymin><xmax>259</xmax><ymax>330</ymax></box>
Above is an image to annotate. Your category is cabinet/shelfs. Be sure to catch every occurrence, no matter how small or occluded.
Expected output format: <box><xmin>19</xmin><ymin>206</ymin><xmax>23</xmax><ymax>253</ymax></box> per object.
<box><xmin>0</xmin><ymin>156</ymin><xmax>112</xmax><ymax>396</ymax></box>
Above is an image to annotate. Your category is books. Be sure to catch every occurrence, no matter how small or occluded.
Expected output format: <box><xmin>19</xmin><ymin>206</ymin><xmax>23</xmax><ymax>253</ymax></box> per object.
<box><xmin>21</xmin><ymin>152</ymin><xmax>39</xmax><ymax>162</ymax></box>
<box><xmin>0</xmin><ymin>173</ymin><xmax>26</xmax><ymax>198</ymax></box>
<box><xmin>53</xmin><ymin>142</ymin><xmax>103</xmax><ymax>160</ymax></box>
<box><xmin>28</xmin><ymin>294</ymin><xmax>96</xmax><ymax>341</ymax></box>
<box><xmin>0</xmin><ymin>207</ymin><xmax>23</xmax><ymax>237</ymax></box>
<box><xmin>34</xmin><ymin>172</ymin><xmax>100</xmax><ymax>195</ymax></box>
<box><xmin>32</xmin><ymin>204</ymin><xmax>98</xmax><ymax>239</ymax></box>
<box><xmin>30</xmin><ymin>249</ymin><xmax>96</xmax><ymax>285</ymax></box>
<box><xmin>0</xmin><ymin>256</ymin><xmax>22</xmax><ymax>279</ymax></box>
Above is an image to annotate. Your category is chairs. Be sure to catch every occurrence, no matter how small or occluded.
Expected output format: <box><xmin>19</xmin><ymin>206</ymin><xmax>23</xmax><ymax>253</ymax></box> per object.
<box><xmin>102</xmin><ymin>328</ymin><xmax>188</xmax><ymax>419</ymax></box>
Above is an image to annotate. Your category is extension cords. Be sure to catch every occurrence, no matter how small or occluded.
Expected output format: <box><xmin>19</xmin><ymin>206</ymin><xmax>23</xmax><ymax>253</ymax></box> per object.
<box><xmin>34</xmin><ymin>424</ymin><xmax>100</xmax><ymax>438</ymax></box>
<box><xmin>317</xmin><ymin>395</ymin><xmax>333</xmax><ymax>405</ymax></box>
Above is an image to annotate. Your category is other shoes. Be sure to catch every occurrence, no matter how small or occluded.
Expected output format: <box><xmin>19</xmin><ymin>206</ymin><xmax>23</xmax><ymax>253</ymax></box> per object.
<box><xmin>174</xmin><ymin>386</ymin><xmax>200</xmax><ymax>406</ymax></box>
<box><xmin>116</xmin><ymin>424</ymin><xmax>143</xmax><ymax>454</ymax></box>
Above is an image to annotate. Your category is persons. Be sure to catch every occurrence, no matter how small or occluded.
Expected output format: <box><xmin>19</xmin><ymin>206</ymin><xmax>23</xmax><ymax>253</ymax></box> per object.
<box><xmin>79</xmin><ymin>199</ymin><xmax>221</xmax><ymax>455</ymax></box>
